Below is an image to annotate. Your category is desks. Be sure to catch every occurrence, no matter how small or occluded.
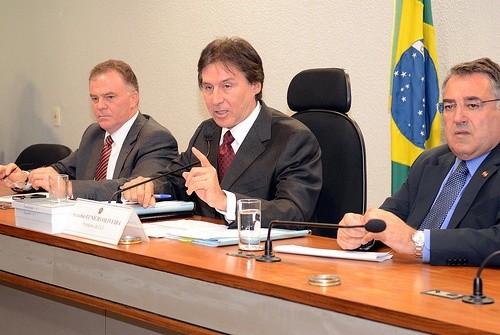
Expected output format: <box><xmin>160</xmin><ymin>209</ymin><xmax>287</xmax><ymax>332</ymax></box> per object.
<box><xmin>0</xmin><ymin>179</ymin><xmax>500</xmax><ymax>335</ymax></box>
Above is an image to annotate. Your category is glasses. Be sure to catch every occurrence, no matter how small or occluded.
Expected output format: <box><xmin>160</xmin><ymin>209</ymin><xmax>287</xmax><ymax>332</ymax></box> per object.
<box><xmin>436</xmin><ymin>97</ymin><xmax>500</xmax><ymax>113</ymax></box>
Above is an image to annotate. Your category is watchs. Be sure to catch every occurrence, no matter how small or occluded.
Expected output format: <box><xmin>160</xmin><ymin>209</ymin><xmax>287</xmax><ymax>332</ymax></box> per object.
<box><xmin>412</xmin><ymin>230</ymin><xmax>424</xmax><ymax>258</ymax></box>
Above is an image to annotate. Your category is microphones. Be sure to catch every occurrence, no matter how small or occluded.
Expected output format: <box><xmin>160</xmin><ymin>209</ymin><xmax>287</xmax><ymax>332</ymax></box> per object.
<box><xmin>2</xmin><ymin>160</ymin><xmax>43</xmax><ymax>180</ymax></box>
<box><xmin>256</xmin><ymin>219</ymin><xmax>386</xmax><ymax>262</ymax></box>
<box><xmin>107</xmin><ymin>129</ymin><xmax>213</xmax><ymax>205</ymax></box>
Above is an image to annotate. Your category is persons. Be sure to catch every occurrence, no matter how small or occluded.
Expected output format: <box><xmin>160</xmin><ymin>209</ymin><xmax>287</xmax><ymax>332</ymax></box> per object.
<box><xmin>123</xmin><ymin>36</ymin><xmax>323</xmax><ymax>235</ymax></box>
<box><xmin>336</xmin><ymin>57</ymin><xmax>500</xmax><ymax>268</ymax></box>
<box><xmin>0</xmin><ymin>60</ymin><xmax>178</xmax><ymax>202</ymax></box>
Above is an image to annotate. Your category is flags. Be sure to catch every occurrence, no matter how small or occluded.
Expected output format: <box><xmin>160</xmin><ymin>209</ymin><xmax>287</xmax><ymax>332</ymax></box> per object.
<box><xmin>390</xmin><ymin>0</ymin><xmax>441</xmax><ymax>196</ymax></box>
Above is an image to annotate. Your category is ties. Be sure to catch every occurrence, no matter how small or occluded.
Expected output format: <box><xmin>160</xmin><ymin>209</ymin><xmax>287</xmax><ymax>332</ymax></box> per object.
<box><xmin>418</xmin><ymin>159</ymin><xmax>470</xmax><ymax>238</ymax></box>
<box><xmin>217</xmin><ymin>130</ymin><xmax>236</xmax><ymax>184</ymax></box>
<box><xmin>95</xmin><ymin>135</ymin><xmax>113</xmax><ymax>181</ymax></box>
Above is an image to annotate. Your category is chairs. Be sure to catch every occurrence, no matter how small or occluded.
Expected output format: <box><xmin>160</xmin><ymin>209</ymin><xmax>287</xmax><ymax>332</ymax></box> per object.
<box><xmin>16</xmin><ymin>143</ymin><xmax>72</xmax><ymax>171</ymax></box>
<box><xmin>287</xmin><ymin>68</ymin><xmax>367</xmax><ymax>238</ymax></box>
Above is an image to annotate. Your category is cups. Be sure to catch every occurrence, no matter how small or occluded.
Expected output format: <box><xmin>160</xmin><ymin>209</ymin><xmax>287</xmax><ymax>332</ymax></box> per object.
<box><xmin>48</xmin><ymin>174</ymin><xmax>68</xmax><ymax>200</ymax></box>
<box><xmin>237</xmin><ymin>199</ymin><xmax>261</xmax><ymax>251</ymax></box>
